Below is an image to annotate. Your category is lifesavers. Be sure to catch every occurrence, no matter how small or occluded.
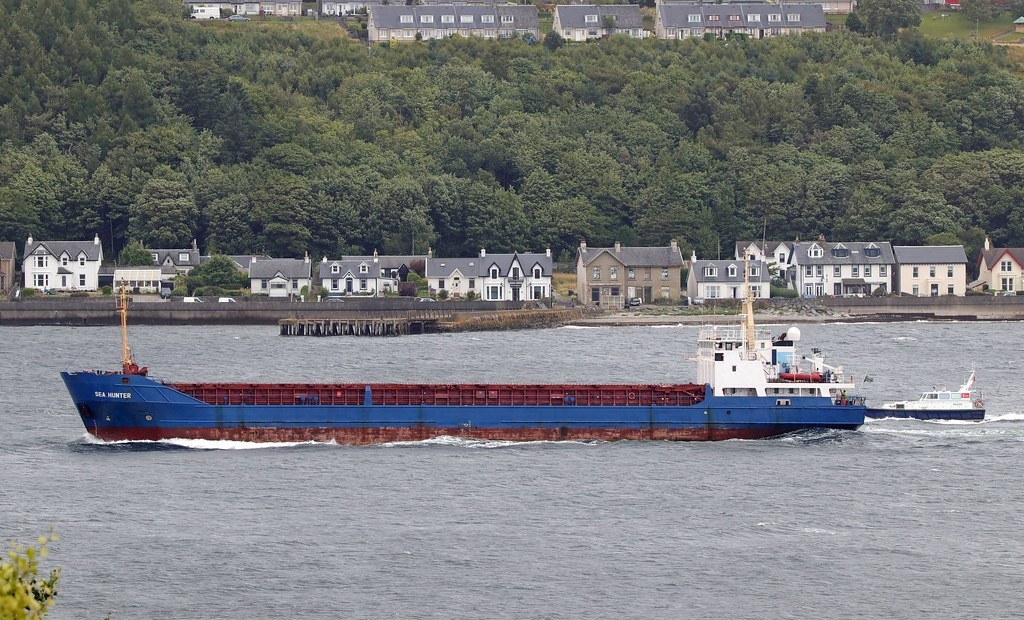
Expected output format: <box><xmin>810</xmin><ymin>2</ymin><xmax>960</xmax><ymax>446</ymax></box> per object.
<box><xmin>977</xmin><ymin>400</ymin><xmax>983</xmax><ymax>406</ymax></box>
<box><xmin>726</xmin><ymin>410</ymin><xmax>731</xmax><ymax>415</ymax></box>
<box><xmin>628</xmin><ymin>392</ymin><xmax>635</xmax><ymax>399</ymax></box>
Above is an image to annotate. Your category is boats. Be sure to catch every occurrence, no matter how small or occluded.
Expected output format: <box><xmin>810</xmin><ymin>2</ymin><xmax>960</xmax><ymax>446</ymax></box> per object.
<box><xmin>61</xmin><ymin>247</ymin><xmax>864</xmax><ymax>439</ymax></box>
<box><xmin>864</xmin><ymin>373</ymin><xmax>986</xmax><ymax>420</ymax></box>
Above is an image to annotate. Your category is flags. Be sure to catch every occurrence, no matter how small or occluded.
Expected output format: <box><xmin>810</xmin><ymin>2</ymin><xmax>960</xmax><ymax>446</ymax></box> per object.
<box><xmin>864</xmin><ymin>375</ymin><xmax>874</xmax><ymax>383</ymax></box>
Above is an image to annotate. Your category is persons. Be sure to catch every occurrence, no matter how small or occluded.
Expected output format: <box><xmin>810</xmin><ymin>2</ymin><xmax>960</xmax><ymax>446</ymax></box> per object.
<box><xmin>840</xmin><ymin>389</ymin><xmax>847</xmax><ymax>405</ymax></box>
<box><xmin>224</xmin><ymin>393</ymin><xmax>228</xmax><ymax>405</ymax></box>
<box><xmin>564</xmin><ymin>392</ymin><xmax>575</xmax><ymax>406</ymax></box>
<box><xmin>300</xmin><ymin>394</ymin><xmax>318</xmax><ymax>405</ymax></box>
